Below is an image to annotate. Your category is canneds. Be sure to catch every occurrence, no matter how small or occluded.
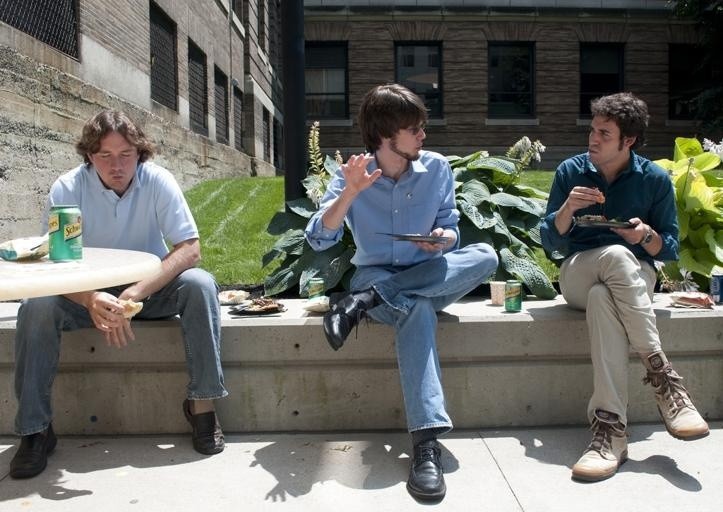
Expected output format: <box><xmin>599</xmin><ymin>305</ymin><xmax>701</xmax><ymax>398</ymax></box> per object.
<box><xmin>308</xmin><ymin>278</ymin><xmax>325</xmax><ymax>301</ymax></box>
<box><xmin>48</xmin><ymin>204</ymin><xmax>84</xmax><ymax>262</ymax></box>
<box><xmin>711</xmin><ymin>274</ymin><xmax>722</xmax><ymax>304</ymax></box>
<box><xmin>505</xmin><ymin>279</ymin><xmax>522</xmax><ymax>313</ymax></box>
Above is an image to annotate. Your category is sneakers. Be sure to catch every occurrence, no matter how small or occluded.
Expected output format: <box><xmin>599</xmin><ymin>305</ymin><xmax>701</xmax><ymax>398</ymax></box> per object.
<box><xmin>643</xmin><ymin>352</ymin><xmax>710</xmax><ymax>441</ymax></box>
<box><xmin>572</xmin><ymin>409</ymin><xmax>627</xmax><ymax>482</ymax></box>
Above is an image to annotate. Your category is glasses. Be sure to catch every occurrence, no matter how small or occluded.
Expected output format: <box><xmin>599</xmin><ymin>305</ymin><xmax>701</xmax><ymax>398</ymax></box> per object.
<box><xmin>404</xmin><ymin>119</ymin><xmax>426</xmax><ymax>135</ymax></box>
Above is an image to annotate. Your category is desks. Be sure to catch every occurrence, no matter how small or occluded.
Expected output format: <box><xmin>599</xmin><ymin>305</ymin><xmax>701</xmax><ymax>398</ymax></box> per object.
<box><xmin>0</xmin><ymin>246</ymin><xmax>164</xmax><ymax>303</ymax></box>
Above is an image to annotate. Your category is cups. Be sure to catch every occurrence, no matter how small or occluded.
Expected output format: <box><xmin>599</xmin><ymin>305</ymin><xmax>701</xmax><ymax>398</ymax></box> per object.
<box><xmin>489</xmin><ymin>281</ymin><xmax>505</xmax><ymax>305</ymax></box>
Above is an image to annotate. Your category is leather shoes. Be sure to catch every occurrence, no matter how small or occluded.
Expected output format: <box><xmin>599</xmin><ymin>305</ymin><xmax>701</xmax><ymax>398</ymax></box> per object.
<box><xmin>11</xmin><ymin>423</ymin><xmax>56</xmax><ymax>479</ymax></box>
<box><xmin>323</xmin><ymin>291</ymin><xmax>367</xmax><ymax>351</ymax></box>
<box><xmin>407</xmin><ymin>438</ymin><xmax>446</xmax><ymax>499</ymax></box>
<box><xmin>183</xmin><ymin>399</ymin><xmax>225</xmax><ymax>454</ymax></box>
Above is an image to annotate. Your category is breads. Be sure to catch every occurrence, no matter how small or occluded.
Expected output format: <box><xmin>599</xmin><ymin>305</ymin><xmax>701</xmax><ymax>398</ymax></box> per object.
<box><xmin>114</xmin><ymin>298</ymin><xmax>144</xmax><ymax>319</ymax></box>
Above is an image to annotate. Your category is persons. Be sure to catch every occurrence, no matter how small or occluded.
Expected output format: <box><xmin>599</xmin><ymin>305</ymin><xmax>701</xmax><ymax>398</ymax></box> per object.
<box><xmin>7</xmin><ymin>108</ymin><xmax>231</xmax><ymax>482</ymax></box>
<box><xmin>537</xmin><ymin>91</ymin><xmax>711</xmax><ymax>481</ymax></box>
<box><xmin>303</xmin><ymin>83</ymin><xmax>501</xmax><ymax>502</ymax></box>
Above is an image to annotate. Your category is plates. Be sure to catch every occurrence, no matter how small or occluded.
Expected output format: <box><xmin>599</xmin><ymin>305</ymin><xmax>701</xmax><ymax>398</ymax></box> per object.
<box><xmin>389</xmin><ymin>235</ymin><xmax>450</xmax><ymax>242</ymax></box>
<box><xmin>573</xmin><ymin>218</ymin><xmax>634</xmax><ymax>229</ymax></box>
<box><xmin>217</xmin><ymin>299</ymin><xmax>247</xmax><ymax>306</ymax></box>
<box><xmin>230</xmin><ymin>301</ymin><xmax>284</xmax><ymax>315</ymax></box>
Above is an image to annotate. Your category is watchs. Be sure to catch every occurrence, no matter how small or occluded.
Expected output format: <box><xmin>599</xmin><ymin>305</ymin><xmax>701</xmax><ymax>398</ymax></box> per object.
<box><xmin>639</xmin><ymin>224</ymin><xmax>654</xmax><ymax>247</ymax></box>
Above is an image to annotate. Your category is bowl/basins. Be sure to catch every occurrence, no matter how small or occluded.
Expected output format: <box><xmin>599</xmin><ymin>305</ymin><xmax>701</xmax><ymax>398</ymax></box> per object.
<box><xmin>0</xmin><ymin>236</ymin><xmax>50</xmax><ymax>261</ymax></box>
